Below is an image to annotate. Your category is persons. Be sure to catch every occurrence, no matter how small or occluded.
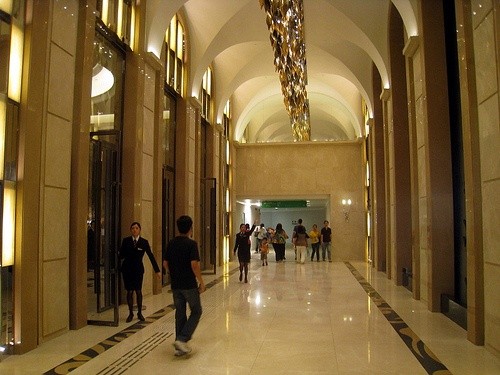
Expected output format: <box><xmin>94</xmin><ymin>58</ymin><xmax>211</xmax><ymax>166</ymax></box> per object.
<box><xmin>163</xmin><ymin>215</ymin><xmax>206</xmax><ymax>357</ymax></box>
<box><xmin>116</xmin><ymin>223</ymin><xmax>161</xmax><ymax>324</ymax></box>
<box><xmin>233</xmin><ymin>218</ymin><xmax>333</xmax><ymax>284</ymax></box>
